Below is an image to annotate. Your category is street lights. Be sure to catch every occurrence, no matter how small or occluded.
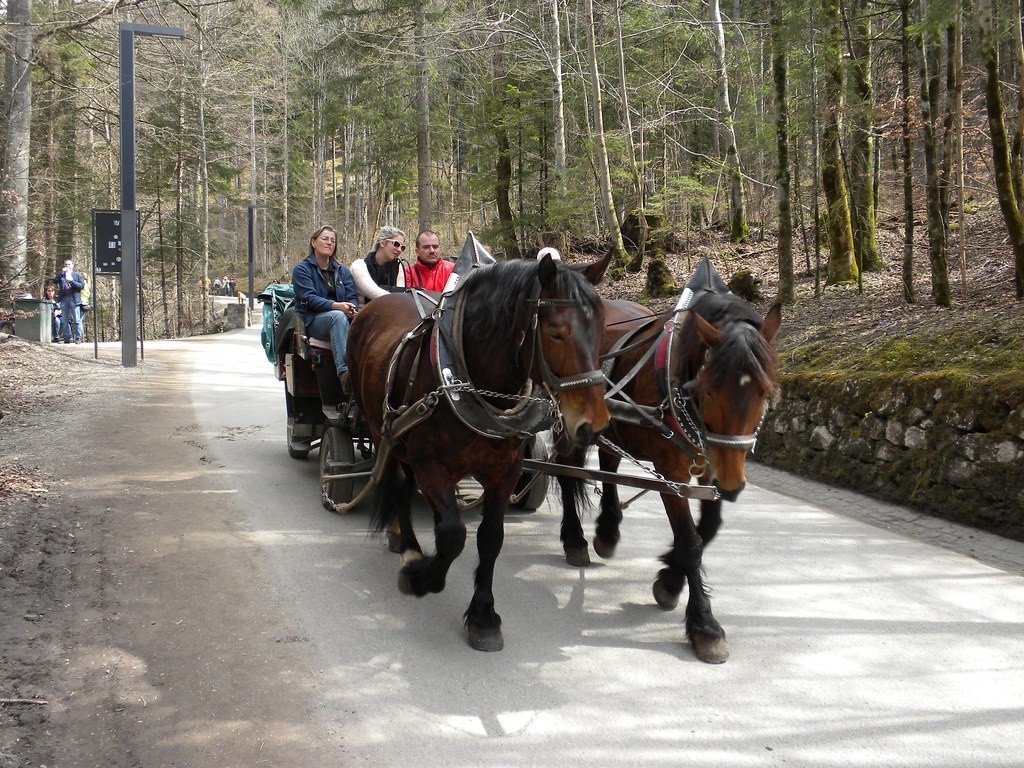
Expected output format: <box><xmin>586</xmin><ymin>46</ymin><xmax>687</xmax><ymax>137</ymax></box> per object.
<box><xmin>119</xmin><ymin>20</ymin><xmax>185</xmax><ymax>367</ymax></box>
<box><xmin>248</xmin><ymin>204</ymin><xmax>270</xmax><ymax>311</ymax></box>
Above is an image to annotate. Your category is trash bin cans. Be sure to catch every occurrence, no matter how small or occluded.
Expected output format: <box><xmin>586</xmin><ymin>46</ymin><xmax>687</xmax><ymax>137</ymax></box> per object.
<box><xmin>14</xmin><ymin>299</ymin><xmax>52</xmax><ymax>343</ymax></box>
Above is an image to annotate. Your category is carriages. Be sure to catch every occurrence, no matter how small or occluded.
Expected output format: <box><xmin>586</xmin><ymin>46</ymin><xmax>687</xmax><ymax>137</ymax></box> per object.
<box><xmin>260</xmin><ymin>231</ymin><xmax>782</xmax><ymax>663</ymax></box>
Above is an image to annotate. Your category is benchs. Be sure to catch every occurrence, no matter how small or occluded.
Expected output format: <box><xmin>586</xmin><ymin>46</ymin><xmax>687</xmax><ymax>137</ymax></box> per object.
<box><xmin>275</xmin><ymin>309</ymin><xmax>349</xmax><ymax>395</ymax></box>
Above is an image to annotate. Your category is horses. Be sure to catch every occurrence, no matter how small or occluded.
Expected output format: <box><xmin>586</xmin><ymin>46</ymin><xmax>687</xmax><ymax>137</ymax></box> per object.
<box><xmin>556</xmin><ymin>295</ymin><xmax>783</xmax><ymax>664</ymax></box>
<box><xmin>344</xmin><ymin>244</ymin><xmax>610</xmax><ymax>651</ymax></box>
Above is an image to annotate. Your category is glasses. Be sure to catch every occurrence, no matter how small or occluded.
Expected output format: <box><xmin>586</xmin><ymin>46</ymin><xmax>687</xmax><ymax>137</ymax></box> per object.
<box><xmin>386</xmin><ymin>239</ymin><xmax>405</xmax><ymax>252</ymax></box>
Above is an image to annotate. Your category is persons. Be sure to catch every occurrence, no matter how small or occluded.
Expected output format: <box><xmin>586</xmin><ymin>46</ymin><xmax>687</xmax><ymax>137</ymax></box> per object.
<box><xmin>53</xmin><ymin>258</ymin><xmax>85</xmax><ymax>344</ymax></box>
<box><xmin>350</xmin><ymin>225</ymin><xmax>407</xmax><ymax>312</ymax></box>
<box><xmin>229</xmin><ymin>278</ymin><xmax>236</xmax><ymax>297</ymax></box>
<box><xmin>12</xmin><ymin>282</ymin><xmax>33</xmax><ymax>309</ymax></box>
<box><xmin>0</xmin><ymin>307</ymin><xmax>15</xmax><ymax>336</ymax></box>
<box><xmin>208</xmin><ymin>275</ymin><xmax>212</xmax><ymax>295</ymax></box>
<box><xmin>45</xmin><ymin>284</ymin><xmax>62</xmax><ymax>344</ymax></box>
<box><xmin>77</xmin><ymin>272</ymin><xmax>92</xmax><ymax>342</ymax></box>
<box><xmin>291</xmin><ymin>225</ymin><xmax>362</xmax><ymax>396</ymax></box>
<box><xmin>214</xmin><ymin>276</ymin><xmax>223</xmax><ymax>296</ymax></box>
<box><xmin>405</xmin><ymin>231</ymin><xmax>455</xmax><ymax>294</ymax></box>
<box><xmin>198</xmin><ymin>277</ymin><xmax>205</xmax><ymax>294</ymax></box>
<box><xmin>222</xmin><ymin>275</ymin><xmax>230</xmax><ymax>296</ymax></box>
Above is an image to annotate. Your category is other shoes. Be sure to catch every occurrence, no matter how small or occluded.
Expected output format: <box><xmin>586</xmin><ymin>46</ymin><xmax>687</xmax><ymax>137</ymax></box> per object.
<box><xmin>340</xmin><ymin>371</ymin><xmax>351</xmax><ymax>395</ymax></box>
